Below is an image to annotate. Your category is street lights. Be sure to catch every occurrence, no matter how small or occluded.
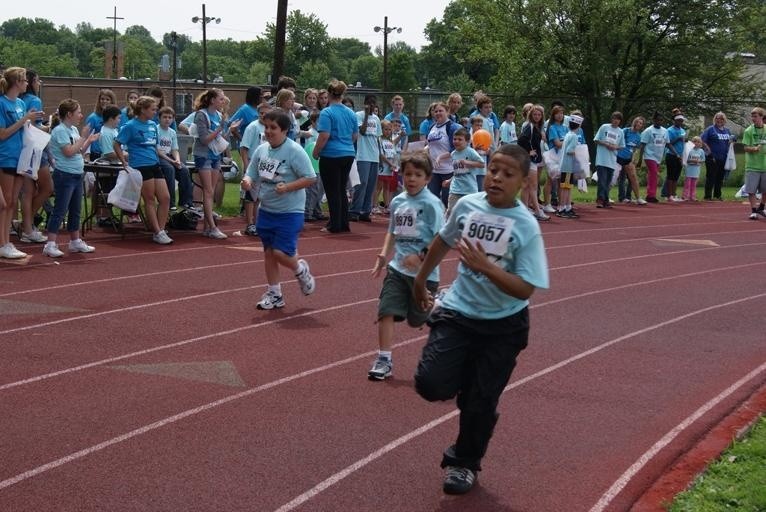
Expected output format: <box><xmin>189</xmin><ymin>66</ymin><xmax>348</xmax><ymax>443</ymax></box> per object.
<box><xmin>373</xmin><ymin>16</ymin><xmax>402</xmax><ymax>117</ymax></box>
<box><xmin>191</xmin><ymin>13</ymin><xmax>222</xmax><ymax>88</ymax></box>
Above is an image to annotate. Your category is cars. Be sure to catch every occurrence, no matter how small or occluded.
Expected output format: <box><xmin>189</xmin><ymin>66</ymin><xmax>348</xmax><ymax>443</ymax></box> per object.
<box><xmin>424</xmin><ymin>86</ymin><xmax>431</xmax><ymax>90</ymax></box>
<box><xmin>408</xmin><ymin>86</ymin><xmax>422</xmax><ymax>127</ymax></box>
<box><xmin>120</xmin><ymin>73</ymin><xmax>224</xmax><ymax>84</ymax></box>
<box><xmin>347</xmin><ymin>83</ymin><xmax>353</xmax><ymax>88</ymax></box>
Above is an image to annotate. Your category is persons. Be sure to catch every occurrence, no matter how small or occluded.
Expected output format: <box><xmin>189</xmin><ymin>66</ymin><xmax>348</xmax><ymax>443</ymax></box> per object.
<box><xmin>241</xmin><ymin>110</ymin><xmax>315</xmax><ymax>310</ymax></box>
<box><xmin>410</xmin><ymin>144</ymin><xmax>550</xmax><ymax>496</ymax></box>
<box><xmin>367</xmin><ymin>148</ymin><xmax>451</xmax><ymax>380</ymax></box>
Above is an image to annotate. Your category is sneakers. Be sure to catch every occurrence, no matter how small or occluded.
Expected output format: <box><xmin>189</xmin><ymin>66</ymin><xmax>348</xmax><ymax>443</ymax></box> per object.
<box><xmin>645</xmin><ymin>196</ymin><xmax>660</xmax><ymax>204</ymax></box>
<box><xmin>595</xmin><ymin>198</ymin><xmax>614</xmax><ymax>209</ymax></box>
<box><xmin>255</xmin><ymin>288</ymin><xmax>286</xmax><ymax>311</ymax></box>
<box><xmin>201</xmin><ymin>226</ymin><xmax>228</xmax><ymax>240</ymax></box>
<box><xmin>637</xmin><ymin>196</ymin><xmax>648</xmax><ymax>205</ymax></box>
<box><xmin>526</xmin><ymin>202</ymin><xmax>579</xmax><ymax>221</ymax></box>
<box><xmin>664</xmin><ymin>193</ymin><xmax>723</xmax><ymax>203</ymax></box>
<box><xmin>757</xmin><ymin>208</ymin><xmax>766</xmax><ymax>217</ymax></box>
<box><xmin>97</xmin><ymin>215</ymin><xmax>120</xmax><ymax>229</ymax></box>
<box><xmin>749</xmin><ymin>211</ymin><xmax>758</xmax><ymax>219</ymax></box>
<box><xmin>319</xmin><ymin>226</ymin><xmax>331</xmax><ymax>234</ymax></box>
<box><xmin>41</xmin><ymin>241</ymin><xmax>65</xmax><ymax>259</ymax></box>
<box><xmin>305</xmin><ymin>215</ymin><xmax>330</xmax><ymax>222</ymax></box>
<box><xmin>622</xmin><ymin>197</ymin><xmax>631</xmax><ymax>204</ymax></box>
<box><xmin>0</xmin><ymin>241</ymin><xmax>28</xmax><ymax>260</ymax></box>
<box><xmin>349</xmin><ymin>215</ymin><xmax>372</xmax><ymax>223</ymax></box>
<box><xmin>292</xmin><ymin>258</ymin><xmax>316</xmax><ymax>297</ymax></box>
<box><xmin>367</xmin><ymin>353</ymin><xmax>394</xmax><ymax>382</ymax></box>
<box><xmin>10</xmin><ymin>219</ymin><xmax>48</xmax><ymax>244</ymax></box>
<box><xmin>442</xmin><ymin>465</ymin><xmax>480</xmax><ymax>496</ymax></box>
<box><xmin>152</xmin><ymin>228</ymin><xmax>175</xmax><ymax>246</ymax></box>
<box><xmin>244</xmin><ymin>223</ymin><xmax>259</xmax><ymax>237</ymax></box>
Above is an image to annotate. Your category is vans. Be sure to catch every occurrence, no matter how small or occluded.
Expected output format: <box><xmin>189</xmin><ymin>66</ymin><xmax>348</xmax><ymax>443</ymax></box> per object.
<box><xmin>731</xmin><ymin>51</ymin><xmax>755</xmax><ymax>64</ymax></box>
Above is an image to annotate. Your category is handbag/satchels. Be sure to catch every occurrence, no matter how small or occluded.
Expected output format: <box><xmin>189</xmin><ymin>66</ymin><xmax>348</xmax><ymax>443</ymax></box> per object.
<box><xmin>15</xmin><ymin>117</ymin><xmax>53</xmax><ymax>183</ymax></box>
<box><xmin>573</xmin><ymin>141</ymin><xmax>591</xmax><ymax>181</ymax></box>
<box><xmin>106</xmin><ymin>164</ymin><xmax>144</xmax><ymax>215</ymax></box>
<box><xmin>541</xmin><ymin>145</ymin><xmax>562</xmax><ymax>184</ymax></box>
<box><xmin>198</xmin><ymin>107</ymin><xmax>230</xmax><ymax>158</ymax></box>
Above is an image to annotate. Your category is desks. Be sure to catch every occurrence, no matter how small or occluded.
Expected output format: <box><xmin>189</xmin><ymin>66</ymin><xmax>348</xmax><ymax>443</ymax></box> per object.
<box><xmin>77</xmin><ymin>161</ymin><xmax>233</xmax><ymax>237</ymax></box>
